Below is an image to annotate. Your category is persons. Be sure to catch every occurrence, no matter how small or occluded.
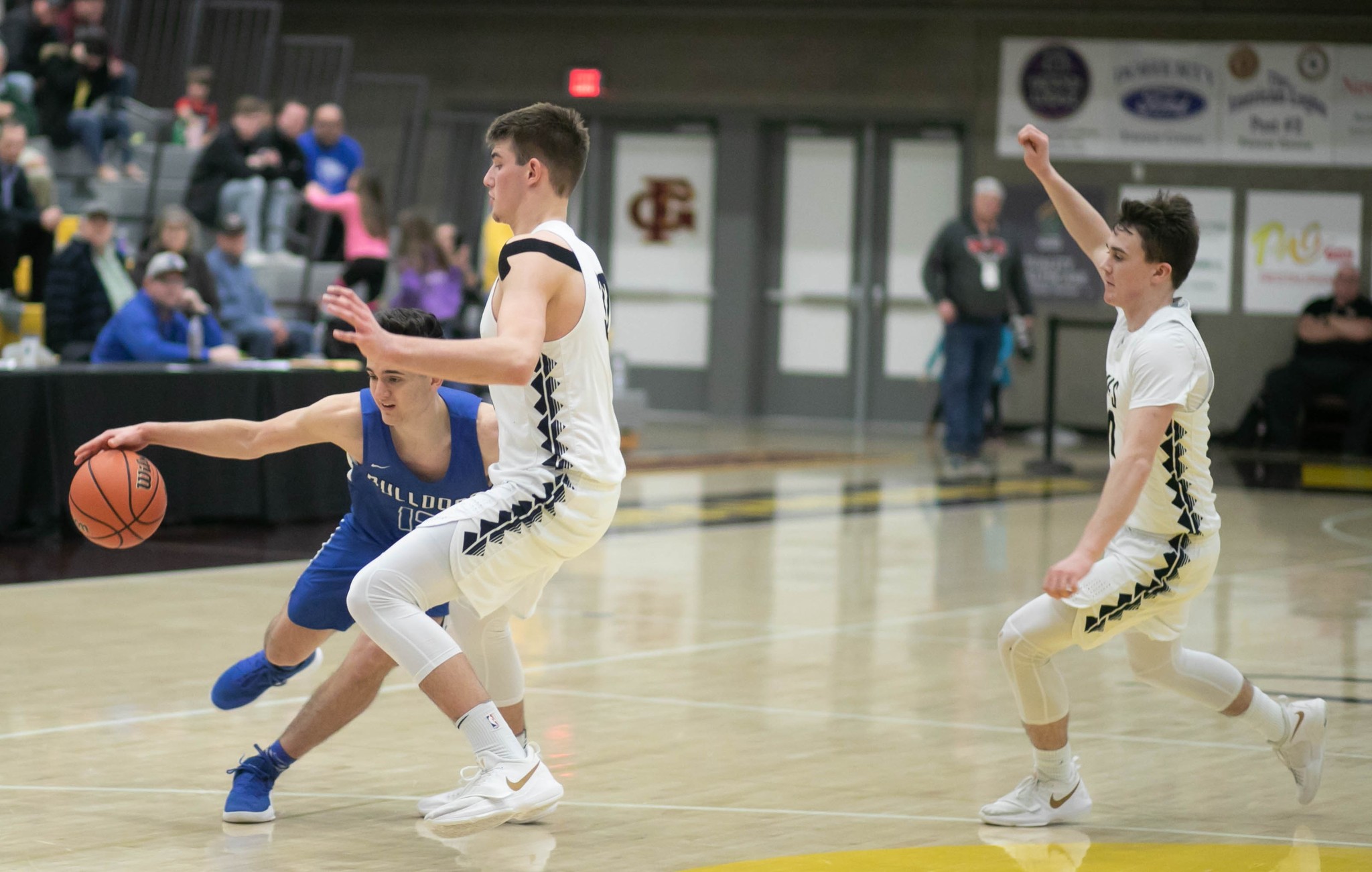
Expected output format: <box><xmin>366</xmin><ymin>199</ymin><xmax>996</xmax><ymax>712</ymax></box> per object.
<box><xmin>977</xmin><ymin>124</ymin><xmax>1328</xmax><ymax>829</ymax></box>
<box><xmin>323</xmin><ymin>103</ymin><xmax>626</xmax><ymax>826</ymax></box>
<box><xmin>1</xmin><ymin>1</ymin><xmax>497</xmax><ymax>364</ymax></box>
<box><xmin>1295</xmin><ymin>258</ymin><xmax>1371</xmax><ymax>463</ymax></box>
<box><xmin>922</xmin><ymin>174</ymin><xmax>1034</xmax><ymax>483</ymax></box>
<box><xmin>73</xmin><ymin>310</ymin><xmax>501</xmax><ymax>823</ymax></box>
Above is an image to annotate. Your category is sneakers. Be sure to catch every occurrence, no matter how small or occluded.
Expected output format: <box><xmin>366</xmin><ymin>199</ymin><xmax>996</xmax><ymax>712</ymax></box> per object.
<box><xmin>210</xmin><ymin>648</ymin><xmax>324</xmax><ymax>712</ymax></box>
<box><xmin>415</xmin><ymin>754</ymin><xmax>556</xmax><ymax>823</ymax></box>
<box><xmin>1268</xmin><ymin>697</ymin><xmax>1328</xmax><ymax>805</ymax></box>
<box><xmin>222</xmin><ymin>755</ymin><xmax>275</xmax><ymax>825</ymax></box>
<box><xmin>412</xmin><ymin>750</ymin><xmax>568</xmax><ymax>840</ymax></box>
<box><xmin>977</xmin><ymin>823</ymin><xmax>1091</xmax><ymax>871</ymax></box>
<box><xmin>980</xmin><ymin>766</ymin><xmax>1093</xmax><ymax>827</ymax></box>
<box><xmin>418</xmin><ymin>818</ymin><xmax>556</xmax><ymax>872</ymax></box>
<box><xmin>221</xmin><ymin>820</ymin><xmax>275</xmax><ymax>855</ymax></box>
<box><xmin>1274</xmin><ymin>824</ymin><xmax>1322</xmax><ymax>872</ymax></box>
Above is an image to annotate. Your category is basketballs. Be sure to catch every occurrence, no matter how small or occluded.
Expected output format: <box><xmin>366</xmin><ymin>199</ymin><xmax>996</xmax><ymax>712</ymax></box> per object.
<box><xmin>68</xmin><ymin>447</ymin><xmax>169</xmax><ymax>550</ymax></box>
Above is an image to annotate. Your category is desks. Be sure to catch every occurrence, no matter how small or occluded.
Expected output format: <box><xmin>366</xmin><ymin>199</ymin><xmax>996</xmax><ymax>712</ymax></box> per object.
<box><xmin>1</xmin><ymin>359</ymin><xmax>368</xmax><ymax>549</ymax></box>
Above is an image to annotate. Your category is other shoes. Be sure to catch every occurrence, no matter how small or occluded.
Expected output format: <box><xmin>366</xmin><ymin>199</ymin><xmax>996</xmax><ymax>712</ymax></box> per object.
<box><xmin>935</xmin><ymin>460</ymin><xmax>1000</xmax><ymax>490</ymax></box>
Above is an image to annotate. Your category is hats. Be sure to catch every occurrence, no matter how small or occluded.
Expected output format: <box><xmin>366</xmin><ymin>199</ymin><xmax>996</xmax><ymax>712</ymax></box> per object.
<box><xmin>144</xmin><ymin>251</ymin><xmax>189</xmax><ymax>281</ymax></box>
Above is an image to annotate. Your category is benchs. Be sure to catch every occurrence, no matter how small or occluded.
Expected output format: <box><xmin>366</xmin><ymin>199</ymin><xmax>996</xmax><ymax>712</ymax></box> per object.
<box><xmin>0</xmin><ymin>99</ymin><xmax>396</xmax><ymax>316</ymax></box>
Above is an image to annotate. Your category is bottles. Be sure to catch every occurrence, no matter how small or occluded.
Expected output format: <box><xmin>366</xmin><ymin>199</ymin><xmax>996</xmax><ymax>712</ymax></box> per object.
<box><xmin>186</xmin><ymin>314</ymin><xmax>204</xmax><ymax>360</ymax></box>
